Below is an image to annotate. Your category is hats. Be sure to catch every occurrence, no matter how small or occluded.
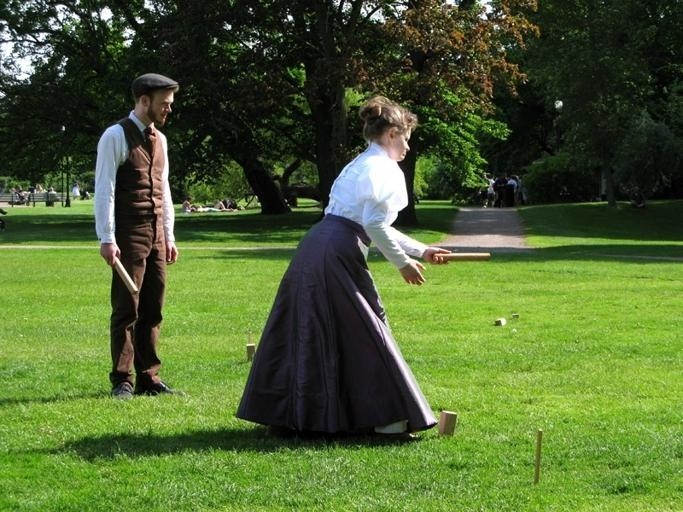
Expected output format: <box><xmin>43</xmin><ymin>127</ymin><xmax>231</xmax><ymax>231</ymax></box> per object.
<box><xmin>131</xmin><ymin>73</ymin><xmax>178</xmax><ymax>96</ymax></box>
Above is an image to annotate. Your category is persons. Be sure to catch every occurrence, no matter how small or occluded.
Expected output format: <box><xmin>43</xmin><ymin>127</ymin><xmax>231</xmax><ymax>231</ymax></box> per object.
<box><xmin>73</xmin><ymin>181</ymin><xmax>80</xmax><ymax>200</ymax></box>
<box><xmin>9</xmin><ymin>184</ymin><xmax>61</xmax><ymax>206</ymax></box>
<box><xmin>236</xmin><ymin>95</ymin><xmax>451</xmax><ymax>440</ymax></box>
<box><xmin>182</xmin><ymin>196</ymin><xmax>237</xmax><ymax>212</ymax></box>
<box><xmin>630</xmin><ymin>187</ymin><xmax>646</xmax><ymax>208</ymax></box>
<box><xmin>95</xmin><ymin>73</ymin><xmax>179</xmax><ymax>399</ymax></box>
<box><xmin>482</xmin><ymin>174</ymin><xmax>527</xmax><ymax>208</ymax></box>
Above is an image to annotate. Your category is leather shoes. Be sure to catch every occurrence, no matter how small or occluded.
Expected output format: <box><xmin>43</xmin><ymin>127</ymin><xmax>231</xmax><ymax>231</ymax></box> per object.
<box><xmin>135</xmin><ymin>382</ymin><xmax>178</xmax><ymax>396</ymax></box>
<box><xmin>109</xmin><ymin>383</ymin><xmax>132</xmax><ymax>401</ymax></box>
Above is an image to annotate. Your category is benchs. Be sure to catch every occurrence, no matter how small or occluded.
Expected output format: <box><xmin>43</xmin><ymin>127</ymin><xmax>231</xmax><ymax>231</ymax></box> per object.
<box><xmin>0</xmin><ymin>192</ymin><xmax>66</xmax><ymax>208</ymax></box>
